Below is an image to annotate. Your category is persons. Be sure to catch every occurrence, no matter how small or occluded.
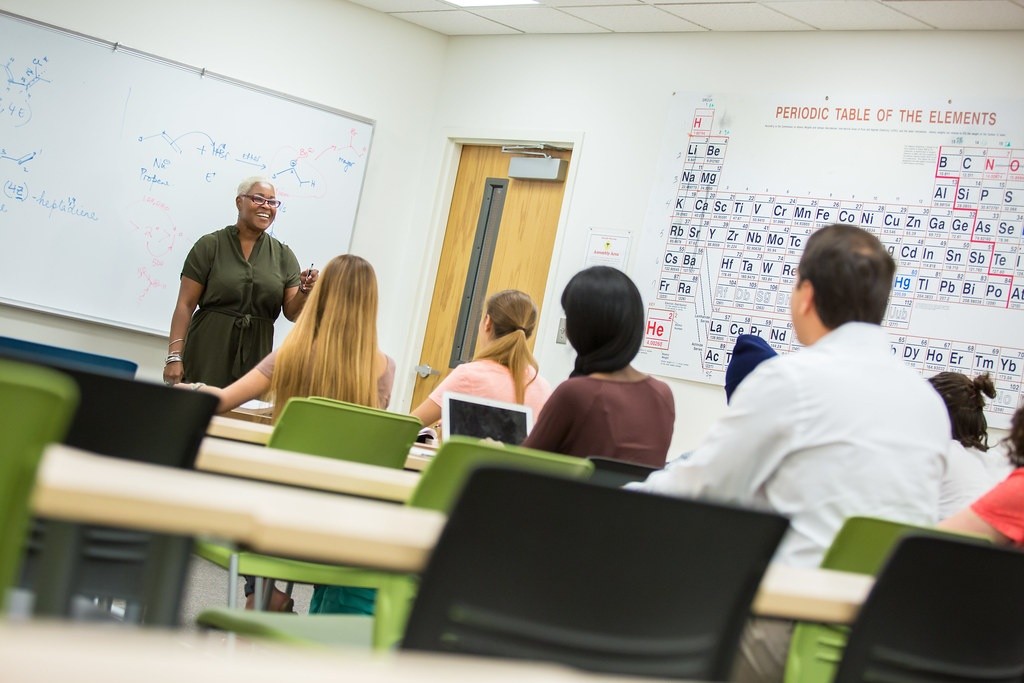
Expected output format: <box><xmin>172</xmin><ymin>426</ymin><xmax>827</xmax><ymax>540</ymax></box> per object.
<box><xmin>475</xmin><ymin>266</ymin><xmax>678</xmax><ymax>480</ymax></box>
<box><xmin>924</xmin><ymin>368</ymin><xmax>1013</xmax><ymax>490</ymax></box>
<box><xmin>619</xmin><ymin>226</ymin><xmax>950</xmax><ymax>562</ymax></box>
<box><xmin>161</xmin><ymin>177</ymin><xmax>320</xmax><ymax>400</ymax></box>
<box><xmin>406</xmin><ymin>288</ymin><xmax>554</xmax><ymax>436</ymax></box>
<box><xmin>173</xmin><ymin>253</ymin><xmax>398</xmax><ymax>422</ymax></box>
<box><xmin>934</xmin><ymin>399</ymin><xmax>1024</xmax><ymax>554</ymax></box>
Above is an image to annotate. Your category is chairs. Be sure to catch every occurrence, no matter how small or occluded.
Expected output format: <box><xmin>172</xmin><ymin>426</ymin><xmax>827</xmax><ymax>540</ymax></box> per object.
<box><xmin>0</xmin><ymin>354</ymin><xmax>1023</xmax><ymax>683</ymax></box>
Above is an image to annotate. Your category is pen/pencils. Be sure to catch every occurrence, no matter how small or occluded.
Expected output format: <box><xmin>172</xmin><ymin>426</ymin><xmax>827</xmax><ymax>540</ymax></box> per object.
<box><xmin>307</xmin><ymin>263</ymin><xmax>314</xmax><ymax>278</ymax></box>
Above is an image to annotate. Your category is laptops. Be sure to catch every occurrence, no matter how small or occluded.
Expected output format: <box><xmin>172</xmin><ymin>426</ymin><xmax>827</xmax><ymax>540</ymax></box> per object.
<box><xmin>442</xmin><ymin>391</ymin><xmax>533</xmax><ymax>445</ymax></box>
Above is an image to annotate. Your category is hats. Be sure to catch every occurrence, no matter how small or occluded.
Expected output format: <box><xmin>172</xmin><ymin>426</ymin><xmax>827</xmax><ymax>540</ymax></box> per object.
<box><xmin>724</xmin><ymin>334</ymin><xmax>778</xmax><ymax>405</ymax></box>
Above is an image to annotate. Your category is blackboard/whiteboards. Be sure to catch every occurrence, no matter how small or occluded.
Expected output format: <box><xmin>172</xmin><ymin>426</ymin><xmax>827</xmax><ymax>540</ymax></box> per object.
<box><xmin>0</xmin><ymin>11</ymin><xmax>376</xmax><ymax>364</ymax></box>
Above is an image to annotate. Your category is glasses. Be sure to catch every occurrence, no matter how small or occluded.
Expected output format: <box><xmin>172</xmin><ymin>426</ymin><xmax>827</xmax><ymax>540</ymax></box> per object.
<box><xmin>241</xmin><ymin>194</ymin><xmax>281</xmax><ymax>209</ymax></box>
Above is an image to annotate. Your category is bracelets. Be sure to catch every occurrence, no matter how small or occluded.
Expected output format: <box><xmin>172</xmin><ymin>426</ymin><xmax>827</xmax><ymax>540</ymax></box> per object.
<box><xmin>165</xmin><ymin>338</ymin><xmax>185</xmax><ymax>364</ymax></box>
<box><xmin>190</xmin><ymin>382</ymin><xmax>205</xmax><ymax>391</ymax></box>
